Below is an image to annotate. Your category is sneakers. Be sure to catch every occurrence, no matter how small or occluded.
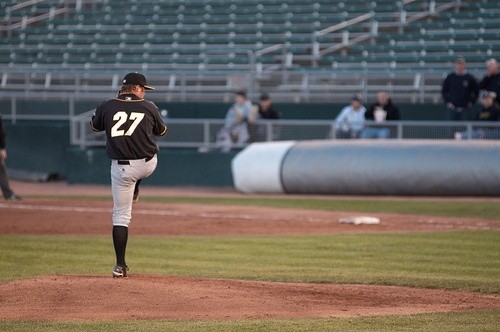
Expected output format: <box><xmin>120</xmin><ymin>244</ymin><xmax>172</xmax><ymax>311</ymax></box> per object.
<box><xmin>113</xmin><ymin>265</ymin><xmax>126</xmax><ymax>276</ymax></box>
<box><xmin>133</xmin><ymin>190</ymin><xmax>139</xmax><ymax>203</ymax></box>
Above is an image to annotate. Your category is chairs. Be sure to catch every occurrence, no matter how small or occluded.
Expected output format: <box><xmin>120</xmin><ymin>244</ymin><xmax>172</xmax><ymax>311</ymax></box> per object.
<box><xmin>0</xmin><ymin>0</ymin><xmax>500</xmax><ymax>101</ymax></box>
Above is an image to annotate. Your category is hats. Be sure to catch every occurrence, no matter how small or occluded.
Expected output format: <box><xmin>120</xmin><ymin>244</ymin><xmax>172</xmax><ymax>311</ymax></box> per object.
<box><xmin>123</xmin><ymin>73</ymin><xmax>155</xmax><ymax>90</ymax></box>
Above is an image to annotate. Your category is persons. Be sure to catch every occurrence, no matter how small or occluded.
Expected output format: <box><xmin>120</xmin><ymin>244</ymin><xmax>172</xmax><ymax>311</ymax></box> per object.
<box><xmin>90</xmin><ymin>72</ymin><xmax>168</xmax><ymax>278</ymax></box>
<box><xmin>442</xmin><ymin>57</ymin><xmax>500</xmax><ymax>136</ymax></box>
<box><xmin>328</xmin><ymin>93</ymin><xmax>402</xmax><ymax>138</ymax></box>
<box><xmin>223</xmin><ymin>92</ymin><xmax>278</xmax><ymax>138</ymax></box>
<box><xmin>0</xmin><ymin>120</ymin><xmax>21</xmax><ymax>201</ymax></box>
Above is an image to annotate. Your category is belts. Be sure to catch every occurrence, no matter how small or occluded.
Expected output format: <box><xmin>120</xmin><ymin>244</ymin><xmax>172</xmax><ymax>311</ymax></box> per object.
<box><xmin>118</xmin><ymin>157</ymin><xmax>153</xmax><ymax>165</ymax></box>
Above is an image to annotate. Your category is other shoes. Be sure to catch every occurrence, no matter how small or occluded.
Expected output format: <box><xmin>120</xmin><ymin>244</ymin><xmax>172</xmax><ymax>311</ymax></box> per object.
<box><xmin>7</xmin><ymin>193</ymin><xmax>23</xmax><ymax>202</ymax></box>
